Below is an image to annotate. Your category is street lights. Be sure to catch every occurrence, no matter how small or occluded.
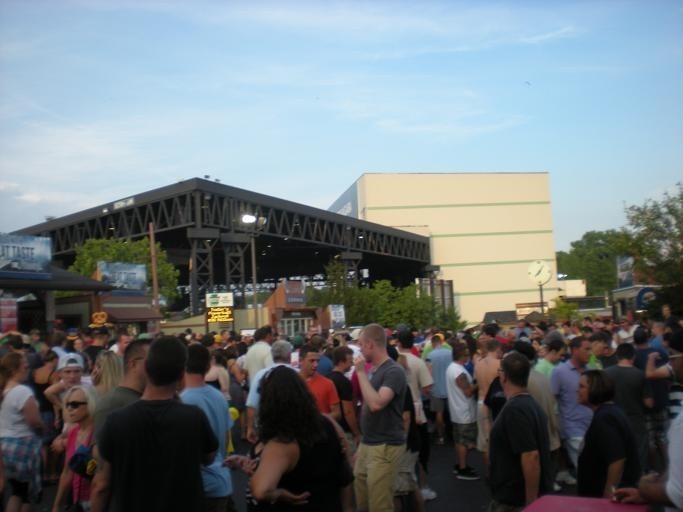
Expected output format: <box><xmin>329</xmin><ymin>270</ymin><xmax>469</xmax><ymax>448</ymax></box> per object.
<box><xmin>240</xmin><ymin>210</ymin><xmax>267</xmax><ymax>330</ymax></box>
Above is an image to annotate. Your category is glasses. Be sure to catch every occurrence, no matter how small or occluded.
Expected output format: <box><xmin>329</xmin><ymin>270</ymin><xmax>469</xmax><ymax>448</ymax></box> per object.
<box><xmin>65</xmin><ymin>401</ymin><xmax>88</xmax><ymax>409</ymax></box>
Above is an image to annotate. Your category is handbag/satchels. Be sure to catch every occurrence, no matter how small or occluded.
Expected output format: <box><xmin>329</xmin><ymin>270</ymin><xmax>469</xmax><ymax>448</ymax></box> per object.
<box><xmin>67</xmin><ymin>444</ymin><xmax>97</xmax><ymax>482</ymax></box>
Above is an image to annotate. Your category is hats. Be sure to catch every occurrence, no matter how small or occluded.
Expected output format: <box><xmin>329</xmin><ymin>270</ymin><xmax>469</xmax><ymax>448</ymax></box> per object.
<box><xmin>391</xmin><ymin>331</ymin><xmax>414</xmax><ymax>344</ymax></box>
<box><xmin>56</xmin><ymin>352</ymin><xmax>84</xmax><ymax>370</ymax></box>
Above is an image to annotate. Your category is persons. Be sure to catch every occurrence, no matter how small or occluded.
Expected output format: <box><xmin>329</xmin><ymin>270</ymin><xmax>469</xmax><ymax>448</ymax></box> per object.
<box><xmin>1</xmin><ymin>298</ymin><xmax>682</xmax><ymax>512</ymax></box>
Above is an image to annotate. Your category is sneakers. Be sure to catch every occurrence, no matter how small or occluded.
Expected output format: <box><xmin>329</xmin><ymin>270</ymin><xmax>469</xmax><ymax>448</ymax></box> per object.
<box><xmin>432</xmin><ymin>437</ymin><xmax>444</xmax><ymax>445</ymax></box>
<box><xmin>454</xmin><ymin>464</ymin><xmax>481</xmax><ymax>481</ymax></box>
<box><xmin>556</xmin><ymin>470</ymin><xmax>577</xmax><ymax>485</ymax></box>
<box><xmin>420</xmin><ymin>488</ymin><xmax>437</xmax><ymax>501</ymax></box>
